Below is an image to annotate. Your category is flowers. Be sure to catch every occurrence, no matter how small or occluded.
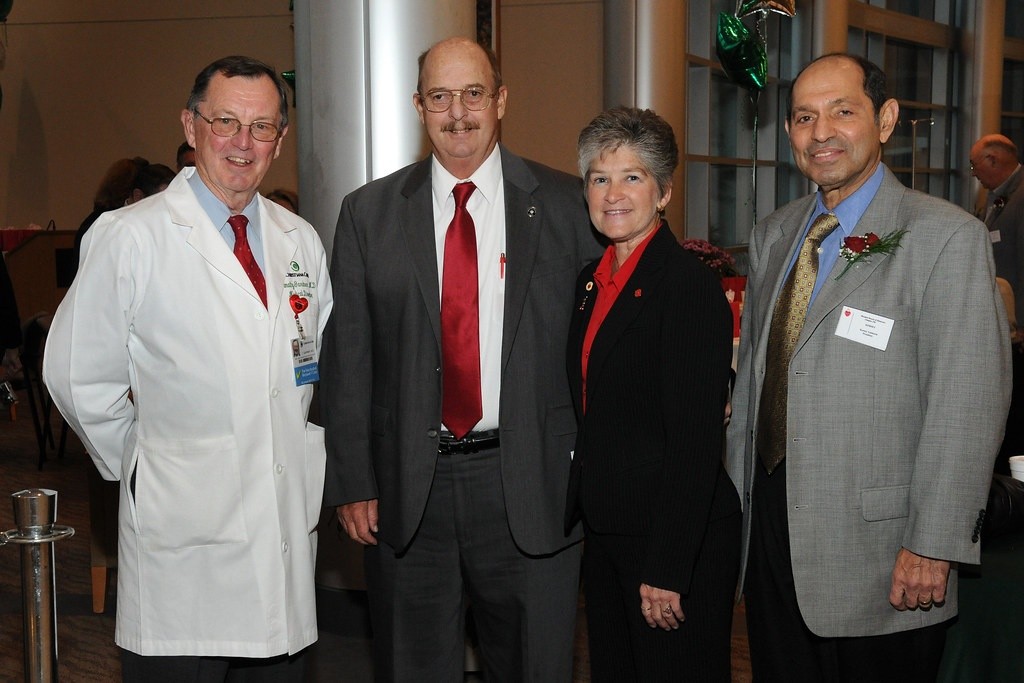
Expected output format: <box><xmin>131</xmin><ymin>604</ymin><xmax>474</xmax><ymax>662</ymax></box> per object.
<box><xmin>834</xmin><ymin>225</ymin><xmax>910</xmax><ymax>283</ymax></box>
<box><xmin>682</xmin><ymin>238</ymin><xmax>736</xmax><ymax>280</ymax></box>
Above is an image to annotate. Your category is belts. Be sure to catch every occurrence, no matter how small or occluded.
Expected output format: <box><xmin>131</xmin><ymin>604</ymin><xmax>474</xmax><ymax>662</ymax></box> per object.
<box><xmin>438</xmin><ymin>438</ymin><xmax>500</xmax><ymax>455</ymax></box>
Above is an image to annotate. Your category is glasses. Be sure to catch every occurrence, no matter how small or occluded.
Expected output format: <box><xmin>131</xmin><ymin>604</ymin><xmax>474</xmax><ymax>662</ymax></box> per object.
<box><xmin>419</xmin><ymin>87</ymin><xmax>497</xmax><ymax>113</ymax></box>
<box><xmin>193</xmin><ymin>108</ymin><xmax>283</xmax><ymax>142</ymax></box>
<box><xmin>969</xmin><ymin>156</ymin><xmax>987</xmax><ymax>172</ymax></box>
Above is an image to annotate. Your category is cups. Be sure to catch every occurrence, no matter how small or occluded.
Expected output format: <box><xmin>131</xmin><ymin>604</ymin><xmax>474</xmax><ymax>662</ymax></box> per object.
<box><xmin>1009</xmin><ymin>456</ymin><xmax>1024</xmax><ymax>482</ymax></box>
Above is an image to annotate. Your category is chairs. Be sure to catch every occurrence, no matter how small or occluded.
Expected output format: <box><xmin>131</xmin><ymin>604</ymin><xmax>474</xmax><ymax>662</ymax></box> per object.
<box><xmin>5</xmin><ymin>231</ymin><xmax>78</xmax><ymax>473</ymax></box>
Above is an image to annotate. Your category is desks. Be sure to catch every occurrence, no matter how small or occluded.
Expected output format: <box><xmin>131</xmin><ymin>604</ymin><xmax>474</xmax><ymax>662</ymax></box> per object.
<box><xmin>718</xmin><ymin>276</ymin><xmax>746</xmax><ymax>336</ymax></box>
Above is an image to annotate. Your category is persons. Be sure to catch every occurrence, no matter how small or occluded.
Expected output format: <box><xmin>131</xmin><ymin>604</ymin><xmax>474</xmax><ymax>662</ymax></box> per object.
<box><xmin>0</xmin><ymin>252</ymin><xmax>24</xmax><ymax>378</ymax></box>
<box><xmin>723</xmin><ymin>52</ymin><xmax>1014</xmax><ymax>683</ymax></box>
<box><xmin>43</xmin><ymin>56</ymin><xmax>334</xmax><ymax>683</ymax></box>
<box><xmin>293</xmin><ymin>340</ymin><xmax>300</xmax><ymax>357</ymax></box>
<box><xmin>314</xmin><ymin>34</ymin><xmax>731</xmax><ymax>682</ymax></box>
<box><xmin>967</xmin><ymin>132</ymin><xmax>1024</xmax><ymax>475</ymax></box>
<box><xmin>74</xmin><ymin>142</ymin><xmax>300</xmax><ymax>272</ymax></box>
<box><xmin>563</xmin><ymin>104</ymin><xmax>737</xmax><ymax>683</ymax></box>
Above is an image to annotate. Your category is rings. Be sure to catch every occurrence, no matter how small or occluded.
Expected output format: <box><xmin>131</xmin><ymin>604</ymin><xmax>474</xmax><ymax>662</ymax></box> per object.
<box><xmin>641</xmin><ymin>606</ymin><xmax>651</xmax><ymax>611</ymax></box>
<box><xmin>662</xmin><ymin>606</ymin><xmax>672</xmax><ymax>614</ymax></box>
<box><xmin>919</xmin><ymin>599</ymin><xmax>932</xmax><ymax>608</ymax></box>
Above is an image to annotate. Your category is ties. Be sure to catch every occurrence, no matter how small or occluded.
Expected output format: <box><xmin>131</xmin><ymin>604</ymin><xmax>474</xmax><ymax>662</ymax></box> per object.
<box><xmin>441</xmin><ymin>180</ymin><xmax>483</xmax><ymax>442</ymax></box>
<box><xmin>756</xmin><ymin>211</ymin><xmax>840</xmax><ymax>477</ymax></box>
<box><xmin>227</xmin><ymin>214</ymin><xmax>268</xmax><ymax>310</ymax></box>
